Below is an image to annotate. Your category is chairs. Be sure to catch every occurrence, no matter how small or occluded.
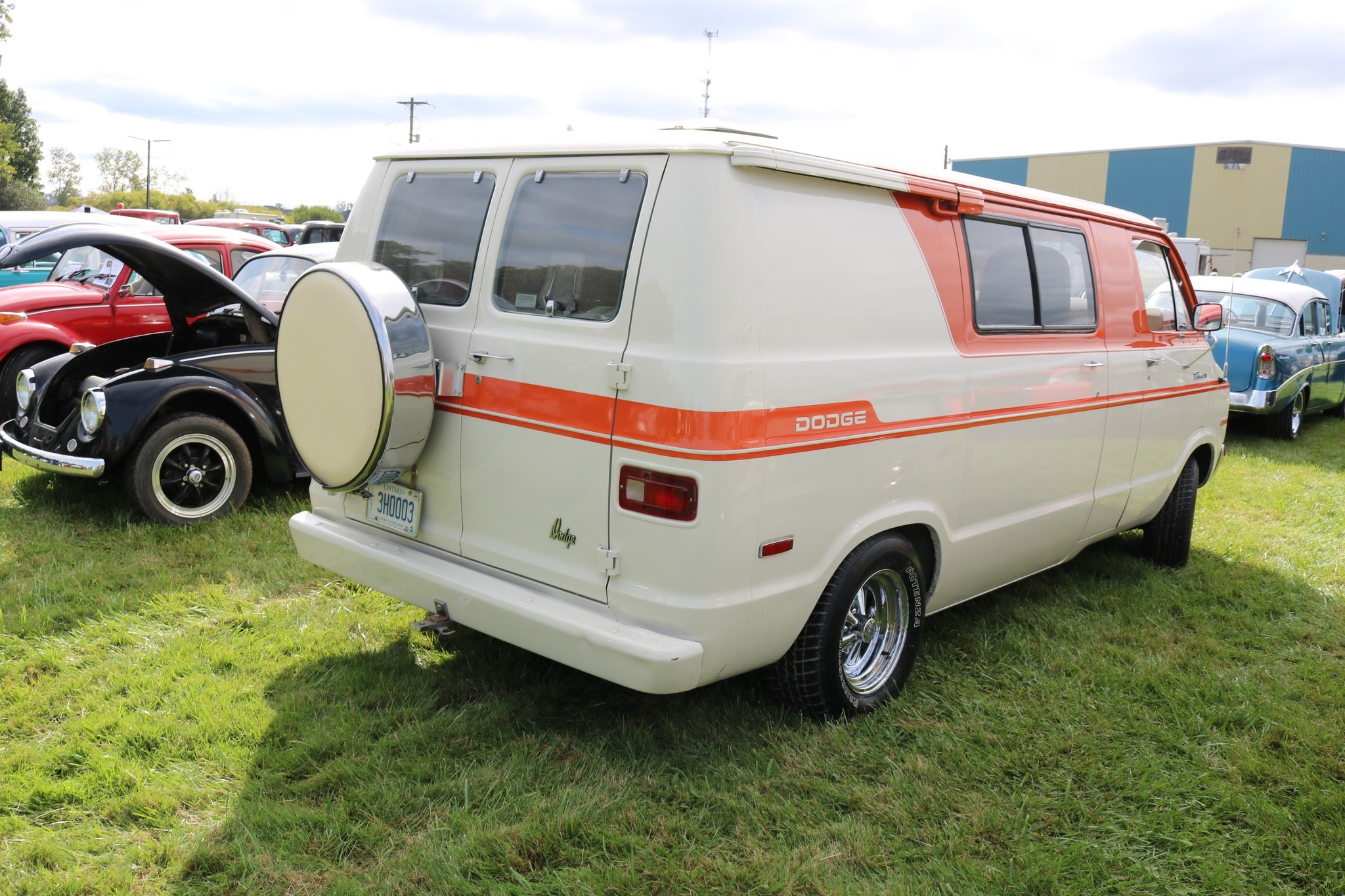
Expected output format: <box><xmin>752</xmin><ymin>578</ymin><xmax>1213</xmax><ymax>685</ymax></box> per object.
<box><xmin>977</xmin><ymin>243</ymin><xmax>1073</xmax><ymax>326</ymax></box>
<box><xmin>408</xmin><ymin>240</ymin><xmax>457</xmax><ymax>300</ymax></box>
<box><xmin>535</xmin><ymin>249</ymin><xmax>616</xmax><ymax>319</ymax></box>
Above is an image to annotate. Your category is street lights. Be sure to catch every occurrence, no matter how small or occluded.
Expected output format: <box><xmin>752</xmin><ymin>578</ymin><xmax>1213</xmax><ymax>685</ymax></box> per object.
<box><xmin>128</xmin><ymin>135</ymin><xmax>172</xmax><ymax>211</ymax></box>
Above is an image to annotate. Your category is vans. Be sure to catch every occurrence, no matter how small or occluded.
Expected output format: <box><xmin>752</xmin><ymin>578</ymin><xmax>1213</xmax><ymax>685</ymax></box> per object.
<box><xmin>273</xmin><ymin>133</ymin><xmax>1239</xmax><ymax>725</ymax></box>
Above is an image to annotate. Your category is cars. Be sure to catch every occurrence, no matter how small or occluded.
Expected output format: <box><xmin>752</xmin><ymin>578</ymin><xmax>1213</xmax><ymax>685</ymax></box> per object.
<box><xmin>1146</xmin><ymin>259</ymin><xmax>1345</xmax><ymax>443</ymax></box>
<box><xmin>0</xmin><ymin>203</ymin><xmax>347</xmax><ymax>531</ymax></box>
